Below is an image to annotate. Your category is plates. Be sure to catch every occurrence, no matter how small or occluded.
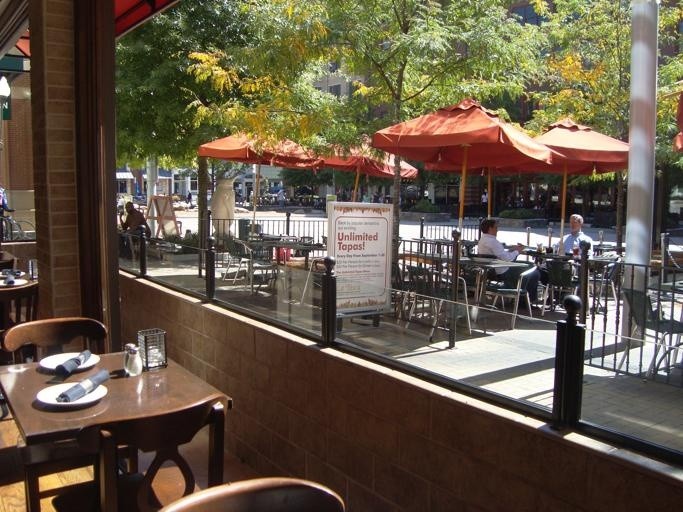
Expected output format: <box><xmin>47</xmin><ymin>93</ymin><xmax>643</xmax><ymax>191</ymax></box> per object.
<box><xmin>0</xmin><ymin>270</ymin><xmax>26</xmax><ymax>277</ymax></box>
<box><xmin>35</xmin><ymin>381</ymin><xmax>109</xmax><ymax>407</ymax></box>
<box><xmin>0</xmin><ymin>278</ymin><xmax>29</xmax><ymax>287</ymax></box>
<box><xmin>38</xmin><ymin>352</ymin><xmax>100</xmax><ymax>371</ymax></box>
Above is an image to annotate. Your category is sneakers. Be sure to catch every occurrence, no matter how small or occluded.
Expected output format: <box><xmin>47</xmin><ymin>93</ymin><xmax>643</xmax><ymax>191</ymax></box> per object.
<box><xmin>521</xmin><ymin>304</ymin><xmax>539</xmax><ymax>310</ymax></box>
<box><xmin>542</xmin><ymin>304</ymin><xmax>553</xmax><ymax>310</ymax></box>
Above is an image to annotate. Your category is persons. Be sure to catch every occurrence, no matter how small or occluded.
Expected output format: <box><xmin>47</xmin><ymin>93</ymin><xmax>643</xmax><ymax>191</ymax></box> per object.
<box><xmin>117</xmin><ymin>202</ymin><xmax>151</xmax><ymax>257</ymax></box>
<box><xmin>549</xmin><ymin>214</ymin><xmax>595</xmax><ymax>306</ymax></box>
<box><xmin>481</xmin><ymin>193</ymin><xmax>487</xmax><ymax>205</ymax></box>
<box><xmin>476</xmin><ymin>219</ymin><xmax>540</xmax><ymax>308</ymax></box>
<box><xmin>185</xmin><ymin>192</ymin><xmax>192</xmax><ymax>203</ymax></box>
<box><xmin>235</xmin><ymin>189</ymin><xmax>317</xmax><ymax>210</ymax></box>
<box><xmin>0</xmin><ymin>188</ymin><xmax>15</xmax><ymax>242</ymax></box>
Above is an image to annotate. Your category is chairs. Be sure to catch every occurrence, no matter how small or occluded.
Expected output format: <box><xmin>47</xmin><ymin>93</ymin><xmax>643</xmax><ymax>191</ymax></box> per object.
<box><xmin>156</xmin><ymin>477</ymin><xmax>346</xmax><ymax>512</ymax></box>
<box><xmin>51</xmin><ymin>393</ymin><xmax>229</xmax><ymax>512</ymax></box>
<box><xmin>539</xmin><ymin>260</ymin><xmax>579</xmax><ymax>317</ymax></box>
<box><xmin>244</xmin><ymin>247</ymin><xmax>277</xmax><ymax>295</ymax></box>
<box><xmin>469</xmin><ymin>254</ymin><xmax>505</xmax><ymax>311</ymax></box>
<box><xmin>3</xmin><ymin>317</ymin><xmax>140</xmax><ymax>512</ymax></box>
<box><xmin>403</xmin><ymin>264</ymin><xmax>474</xmax><ymax>342</ymax></box>
<box><xmin>220</xmin><ymin>237</ymin><xmax>254</xmax><ymax>287</ymax></box>
<box><xmin>573</xmin><ymin>256</ymin><xmax>621</xmax><ymax>312</ymax></box>
<box><xmin>300</xmin><ymin>256</ymin><xmax>335</xmax><ymax>305</ymax></box>
<box><xmin>478</xmin><ymin>263</ymin><xmax>538</xmax><ymax>330</ymax></box>
<box><xmin>614</xmin><ymin>287</ymin><xmax>683</xmax><ymax>382</ymax></box>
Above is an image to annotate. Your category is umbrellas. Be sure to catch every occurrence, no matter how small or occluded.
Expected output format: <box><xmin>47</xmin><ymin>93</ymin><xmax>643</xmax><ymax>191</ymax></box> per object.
<box><xmin>318</xmin><ymin>135</ymin><xmax>418</xmax><ymax>202</ymax></box>
<box><xmin>372</xmin><ymin>96</ymin><xmax>566</xmax><ymax>241</ymax></box>
<box><xmin>198</xmin><ymin>126</ymin><xmax>324</xmax><ymax>284</ymax></box>
<box><xmin>424</xmin><ymin>161</ymin><xmax>516</xmax><ymax>219</ymax></box>
<box><xmin>535</xmin><ymin>117</ymin><xmax>629</xmax><ymax>306</ymax></box>
<box><xmin>673</xmin><ymin>92</ymin><xmax>683</xmax><ymax>151</ymax></box>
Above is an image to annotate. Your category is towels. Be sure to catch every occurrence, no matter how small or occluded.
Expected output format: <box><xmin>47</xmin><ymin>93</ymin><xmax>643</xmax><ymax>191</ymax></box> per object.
<box><xmin>299</xmin><ymin>243</ymin><xmax>323</xmax><ymax>247</ymax></box>
<box><xmin>1</xmin><ymin>269</ymin><xmax>20</xmax><ymax>276</ymax></box>
<box><xmin>263</xmin><ymin>236</ymin><xmax>281</xmax><ymax>241</ymax></box>
<box><xmin>4</xmin><ymin>275</ymin><xmax>14</xmax><ymax>284</ymax></box>
<box><xmin>469</xmin><ymin>254</ymin><xmax>497</xmax><ymax>259</ymax></box>
<box><xmin>56</xmin><ymin>350</ymin><xmax>91</xmax><ymax>378</ymax></box>
<box><xmin>57</xmin><ymin>369</ymin><xmax>110</xmax><ymax>402</ymax></box>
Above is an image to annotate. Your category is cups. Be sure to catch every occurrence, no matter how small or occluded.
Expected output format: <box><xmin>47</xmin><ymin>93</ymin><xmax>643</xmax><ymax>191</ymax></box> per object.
<box><xmin>573</xmin><ymin>247</ymin><xmax>579</xmax><ymax>256</ymax></box>
